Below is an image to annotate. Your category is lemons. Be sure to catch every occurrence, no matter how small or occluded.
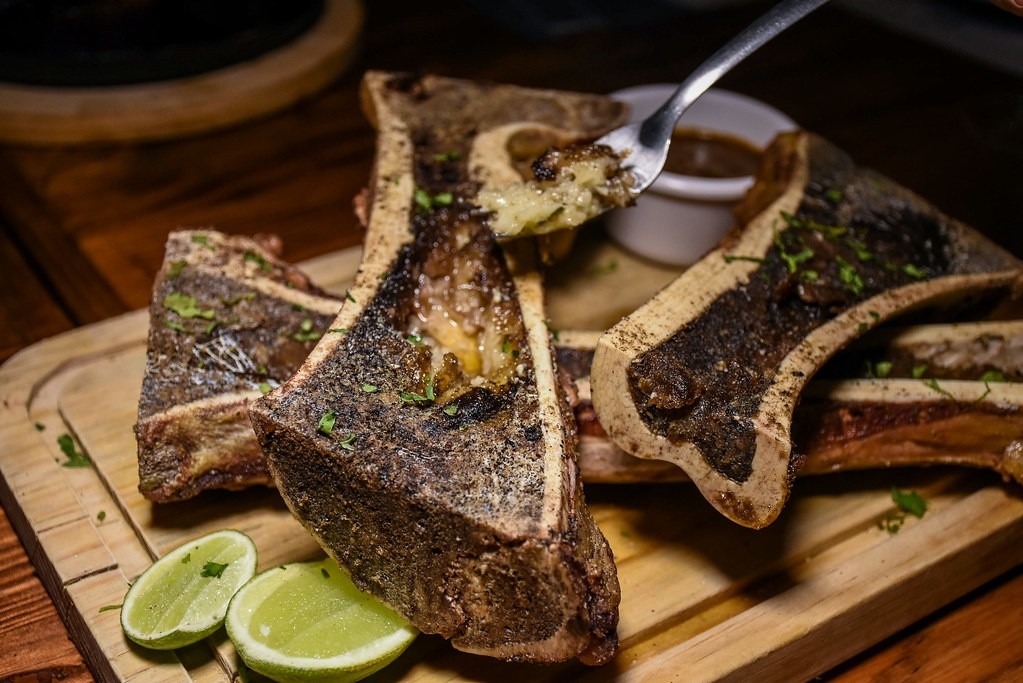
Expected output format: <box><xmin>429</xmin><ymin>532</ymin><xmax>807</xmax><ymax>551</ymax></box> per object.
<box><xmin>120</xmin><ymin>529</ymin><xmax>257</xmax><ymax>650</ymax></box>
<box><xmin>224</xmin><ymin>557</ymin><xmax>419</xmax><ymax>683</ymax></box>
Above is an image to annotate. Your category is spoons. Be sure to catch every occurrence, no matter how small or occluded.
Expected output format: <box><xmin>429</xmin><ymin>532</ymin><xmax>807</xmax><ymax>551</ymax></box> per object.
<box><xmin>486</xmin><ymin>0</ymin><xmax>826</xmax><ymax>232</ymax></box>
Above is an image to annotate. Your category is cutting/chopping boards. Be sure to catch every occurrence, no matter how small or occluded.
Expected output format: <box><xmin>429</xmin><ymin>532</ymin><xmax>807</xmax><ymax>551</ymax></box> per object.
<box><xmin>0</xmin><ymin>171</ymin><xmax>1023</xmax><ymax>683</ymax></box>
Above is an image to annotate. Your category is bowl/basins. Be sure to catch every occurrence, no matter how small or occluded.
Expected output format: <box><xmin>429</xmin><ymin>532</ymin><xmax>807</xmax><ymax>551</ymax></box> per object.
<box><xmin>602</xmin><ymin>84</ymin><xmax>798</xmax><ymax>268</ymax></box>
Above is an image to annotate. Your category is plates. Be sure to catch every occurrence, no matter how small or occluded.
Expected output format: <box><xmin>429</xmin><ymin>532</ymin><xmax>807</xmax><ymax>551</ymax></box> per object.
<box><xmin>0</xmin><ymin>1</ymin><xmax>364</xmax><ymax>143</ymax></box>
